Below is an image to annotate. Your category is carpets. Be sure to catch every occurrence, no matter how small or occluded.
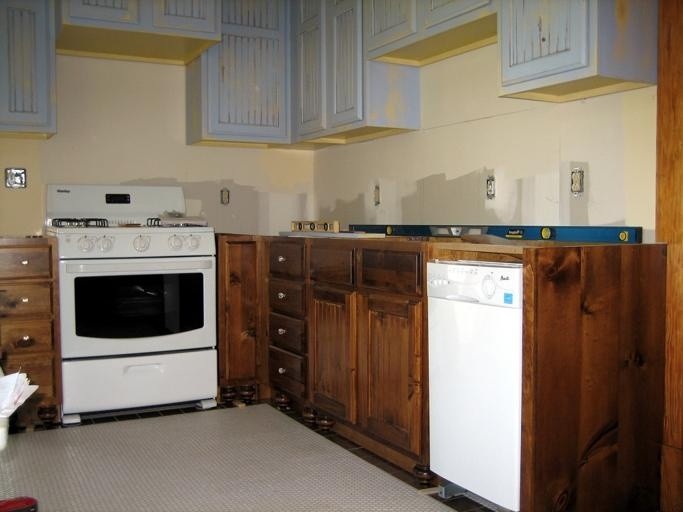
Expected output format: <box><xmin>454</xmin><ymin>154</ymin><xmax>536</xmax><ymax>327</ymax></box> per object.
<box><xmin>0</xmin><ymin>401</ymin><xmax>456</xmax><ymax>511</ymax></box>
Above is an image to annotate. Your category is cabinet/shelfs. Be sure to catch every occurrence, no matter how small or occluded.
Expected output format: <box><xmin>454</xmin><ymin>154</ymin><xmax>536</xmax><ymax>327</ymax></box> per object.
<box><xmin>1</xmin><ymin>234</ymin><xmax>62</xmax><ymax>427</ymax></box>
<box><xmin>266</xmin><ymin>236</ymin><xmax>307</xmax><ymax>421</ymax></box>
<box><xmin>53</xmin><ymin>1</ymin><xmax>221</xmax><ymax>66</ymax></box>
<box><xmin>292</xmin><ymin>1</ymin><xmax>421</xmax><ymax>147</ymax></box>
<box><xmin>0</xmin><ymin>0</ymin><xmax>57</xmax><ymax>140</ymax></box>
<box><xmin>216</xmin><ymin>231</ymin><xmax>266</xmax><ymax>402</ymax></box>
<box><xmin>361</xmin><ymin>0</ymin><xmax>495</xmax><ymax>67</ymax></box>
<box><xmin>306</xmin><ymin>238</ymin><xmax>429</xmax><ymax>481</ymax></box>
<box><xmin>181</xmin><ymin>1</ymin><xmax>292</xmax><ymax>150</ymax></box>
<box><xmin>496</xmin><ymin>0</ymin><xmax>656</xmax><ymax>102</ymax></box>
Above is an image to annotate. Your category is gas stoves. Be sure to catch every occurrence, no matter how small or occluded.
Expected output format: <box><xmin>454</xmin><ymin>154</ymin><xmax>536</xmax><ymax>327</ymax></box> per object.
<box><xmin>47</xmin><ymin>214</ymin><xmax>216</xmax><ymax>256</ymax></box>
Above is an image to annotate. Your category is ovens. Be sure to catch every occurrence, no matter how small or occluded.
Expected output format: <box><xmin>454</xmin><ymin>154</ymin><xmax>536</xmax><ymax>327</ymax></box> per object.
<box><xmin>59</xmin><ymin>259</ymin><xmax>218</xmax><ymax>426</ymax></box>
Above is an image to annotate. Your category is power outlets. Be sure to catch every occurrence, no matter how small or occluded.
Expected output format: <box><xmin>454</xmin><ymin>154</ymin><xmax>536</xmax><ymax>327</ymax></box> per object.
<box><xmin>567</xmin><ymin>168</ymin><xmax>584</xmax><ymax>194</ymax></box>
